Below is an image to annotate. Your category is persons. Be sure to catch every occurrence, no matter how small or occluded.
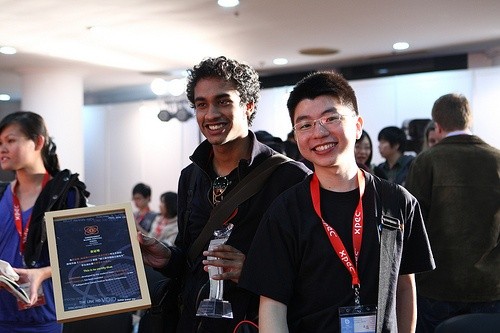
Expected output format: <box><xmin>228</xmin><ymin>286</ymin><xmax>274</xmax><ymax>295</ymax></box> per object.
<box><xmin>237</xmin><ymin>71</ymin><xmax>436</xmax><ymax>333</ymax></box>
<box><xmin>138</xmin><ymin>57</ymin><xmax>314</xmax><ymax>333</ymax></box>
<box><xmin>0</xmin><ymin>110</ymin><xmax>90</xmax><ymax>333</ymax></box>
<box><xmin>254</xmin><ymin>119</ymin><xmax>434</xmax><ymax>186</ymax></box>
<box><xmin>405</xmin><ymin>94</ymin><xmax>500</xmax><ymax>333</ymax></box>
<box><xmin>128</xmin><ymin>183</ymin><xmax>180</xmax><ymax>326</ymax></box>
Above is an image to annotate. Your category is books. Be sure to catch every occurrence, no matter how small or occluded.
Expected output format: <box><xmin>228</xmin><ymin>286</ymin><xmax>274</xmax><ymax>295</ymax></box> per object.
<box><xmin>0</xmin><ymin>275</ymin><xmax>31</xmax><ymax>304</ymax></box>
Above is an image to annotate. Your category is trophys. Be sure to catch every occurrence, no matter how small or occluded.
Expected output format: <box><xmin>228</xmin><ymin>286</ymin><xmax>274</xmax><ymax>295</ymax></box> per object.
<box><xmin>196</xmin><ymin>224</ymin><xmax>234</xmax><ymax>319</ymax></box>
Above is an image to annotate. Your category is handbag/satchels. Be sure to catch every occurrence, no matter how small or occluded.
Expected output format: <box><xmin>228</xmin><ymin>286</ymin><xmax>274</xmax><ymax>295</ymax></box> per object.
<box><xmin>138</xmin><ymin>279</ymin><xmax>182</xmax><ymax>333</ymax></box>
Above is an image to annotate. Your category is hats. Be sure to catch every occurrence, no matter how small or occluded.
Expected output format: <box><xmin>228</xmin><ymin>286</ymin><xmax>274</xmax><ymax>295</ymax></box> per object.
<box><xmin>254</xmin><ymin>130</ymin><xmax>282</xmax><ymax>143</ymax></box>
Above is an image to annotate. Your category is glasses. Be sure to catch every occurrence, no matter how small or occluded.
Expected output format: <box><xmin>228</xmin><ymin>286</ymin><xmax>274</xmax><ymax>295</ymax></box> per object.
<box><xmin>293</xmin><ymin>114</ymin><xmax>355</xmax><ymax>133</ymax></box>
